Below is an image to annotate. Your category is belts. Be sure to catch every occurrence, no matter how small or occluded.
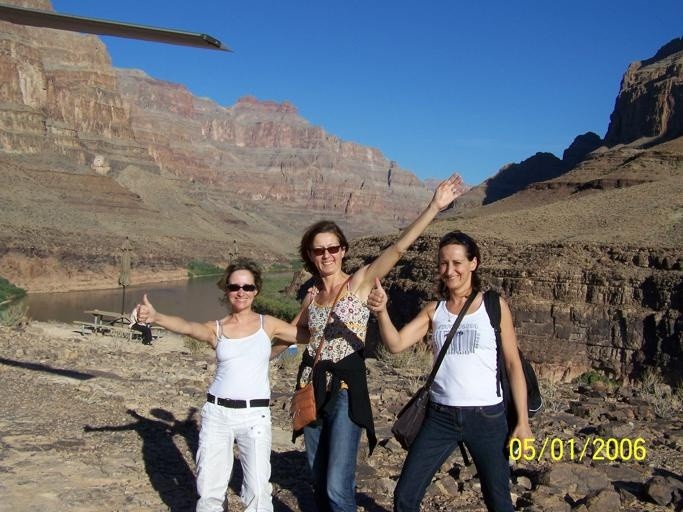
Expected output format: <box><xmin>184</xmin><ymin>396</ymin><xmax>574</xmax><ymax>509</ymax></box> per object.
<box><xmin>206</xmin><ymin>393</ymin><xmax>268</xmax><ymax>408</ymax></box>
<box><xmin>300</xmin><ymin>382</ymin><xmax>348</xmax><ymax>392</ymax></box>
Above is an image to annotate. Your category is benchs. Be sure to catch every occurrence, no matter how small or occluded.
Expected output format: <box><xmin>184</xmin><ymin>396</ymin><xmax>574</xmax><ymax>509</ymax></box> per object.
<box><xmin>72</xmin><ymin>309</ymin><xmax>164</xmax><ymax>342</ymax></box>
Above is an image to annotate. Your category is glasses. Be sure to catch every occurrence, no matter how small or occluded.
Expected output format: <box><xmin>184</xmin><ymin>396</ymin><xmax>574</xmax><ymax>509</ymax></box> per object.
<box><xmin>440</xmin><ymin>233</ymin><xmax>475</xmax><ymax>246</ymax></box>
<box><xmin>309</xmin><ymin>245</ymin><xmax>341</xmax><ymax>255</ymax></box>
<box><xmin>225</xmin><ymin>284</ymin><xmax>255</xmax><ymax>291</ymax></box>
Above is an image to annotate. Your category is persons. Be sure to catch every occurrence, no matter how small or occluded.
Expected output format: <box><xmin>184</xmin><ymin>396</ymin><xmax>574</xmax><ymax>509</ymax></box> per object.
<box><xmin>134</xmin><ymin>258</ymin><xmax>309</xmax><ymax>512</ymax></box>
<box><xmin>128</xmin><ymin>303</ymin><xmax>153</xmax><ymax>345</ymax></box>
<box><xmin>364</xmin><ymin>228</ymin><xmax>535</xmax><ymax>510</ymax></box>
<box><xmin>268</xmin><ymin>172</ymin><xmax>470</xmax><ymax>509</ymax></box>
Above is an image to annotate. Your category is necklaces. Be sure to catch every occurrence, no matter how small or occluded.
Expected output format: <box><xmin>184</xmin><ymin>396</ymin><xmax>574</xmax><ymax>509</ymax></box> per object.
<box><xmin>446</xmin><ymin>304</ymin><xmax>458</xmax><ymax>322</ymax></box>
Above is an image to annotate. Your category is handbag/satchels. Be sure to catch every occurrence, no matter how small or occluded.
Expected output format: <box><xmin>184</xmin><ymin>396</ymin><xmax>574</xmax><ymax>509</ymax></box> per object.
<box><xmin>390</xmin><ymin>384</ymin><xmax>429</xmax><ymax>449</ymax></box>
<box><xmin>497</xmin><ymin>347</ymin><xmax>541</xmax><ymax>426</ymax></box>
<box><xmin>290</xmin><ymin>380</ymin><xmax>317</xmax><ymax>432</ymax></box>
